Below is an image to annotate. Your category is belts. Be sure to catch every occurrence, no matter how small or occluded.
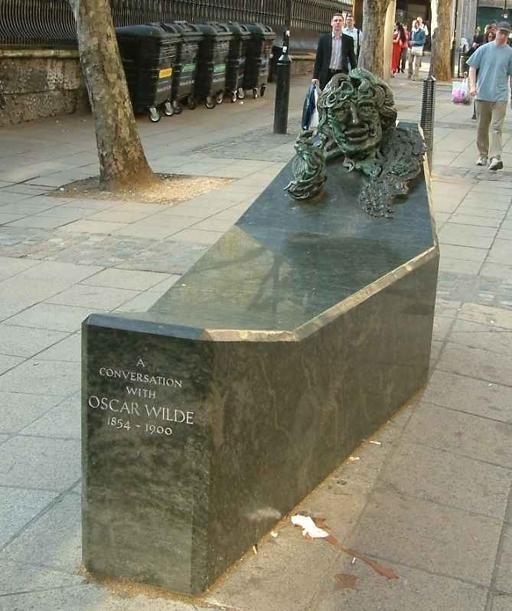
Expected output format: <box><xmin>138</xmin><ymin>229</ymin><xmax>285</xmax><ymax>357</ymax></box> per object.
<box><xmin>329</xmin><ymin>70</ymin><xmax>342</xmax><ymax>74</ymax></box>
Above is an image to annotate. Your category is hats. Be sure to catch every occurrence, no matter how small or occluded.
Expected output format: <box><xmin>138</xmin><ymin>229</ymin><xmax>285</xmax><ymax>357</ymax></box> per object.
<box><xmin>498</xmin><ymin>21</ymin><xmax>511</xmax><ymax>32</ymax></box>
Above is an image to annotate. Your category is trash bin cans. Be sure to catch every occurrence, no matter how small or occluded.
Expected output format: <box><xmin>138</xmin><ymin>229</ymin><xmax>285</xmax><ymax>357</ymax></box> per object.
<box><xmin>459</xmin><ymin>52</ymin><xmax>468</xmax><ymax>78</ymax></box>
<box><xmin>114</xmin><ymin>20</ymin><xmax>276</xmax><ymax>122</ymax></box>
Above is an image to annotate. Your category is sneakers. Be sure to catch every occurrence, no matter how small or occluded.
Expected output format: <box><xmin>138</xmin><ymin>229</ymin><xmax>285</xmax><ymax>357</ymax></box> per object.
<box><xmin>488</xmin><ymin>158</ymin><xmax>503</xmax><ymax>169</ymax></box>
<box><xmin>476</xmin><ymin>156</ymin><xmax>488</xmax><ymax>166</ymax></box>
<box><xmin>472</xmin><ymin>115</ymin><xmax>476</xmax><ymax>121</ymax></box>
<box><xmin>392</xmin><ymin>69</ymin><xmax>418</xmax><ymax>82</ymax></box>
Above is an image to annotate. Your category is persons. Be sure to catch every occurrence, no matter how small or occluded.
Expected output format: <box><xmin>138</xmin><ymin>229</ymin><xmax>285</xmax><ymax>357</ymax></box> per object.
<box><xmin>283</xmin><ymin>65</ymin><xmax>397</xmax><ymax>201</ymax></box>
<box><xmin>458</xmin><ymin>21</ymin><xmax>511</xmax><ymax>170</ymax></box>
<box><xmin>311</xmin><ymin>11</ymin><xmax>363</xmax><ymax>94</ymax></box>
<box><xmin>392</xmin><ymin>16</ymin><xmax>430</xmax><ymax>80</ymax></box>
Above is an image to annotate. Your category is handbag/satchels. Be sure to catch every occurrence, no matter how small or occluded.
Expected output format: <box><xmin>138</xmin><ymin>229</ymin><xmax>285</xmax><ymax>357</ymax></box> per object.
<box><xmin>411</xmin><ymin>45</ymin><xmax>425</xmax><ymax>56</ymax></box>
<box><xmin>302</xmin><ymin>83</ymin><xmax>315</xmax><ymax>130</ymax></box>
